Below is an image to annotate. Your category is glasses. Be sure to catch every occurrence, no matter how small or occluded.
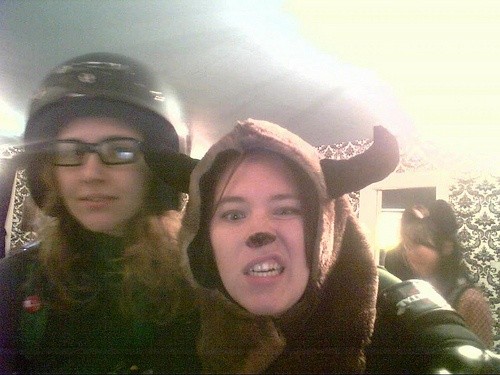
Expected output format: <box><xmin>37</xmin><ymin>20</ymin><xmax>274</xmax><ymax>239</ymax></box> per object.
<box><xmin>37</xmin><ymin>135</ymin><xmax>152</xmax><ymax>168</ymax></box>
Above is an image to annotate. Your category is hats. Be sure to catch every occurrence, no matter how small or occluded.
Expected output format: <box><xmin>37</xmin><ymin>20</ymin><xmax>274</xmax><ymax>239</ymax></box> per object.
<box><xmin>142</xmin><ymin>118</ymin><xmax>400</xmax><ymax>374</ymax></box>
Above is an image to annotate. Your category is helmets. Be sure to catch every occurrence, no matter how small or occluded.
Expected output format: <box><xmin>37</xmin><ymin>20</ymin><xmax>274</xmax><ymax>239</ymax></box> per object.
<box><xmin>22</xmin><ymin>52</ymin><xmax>191</xmax><ymax>217</ymax></box>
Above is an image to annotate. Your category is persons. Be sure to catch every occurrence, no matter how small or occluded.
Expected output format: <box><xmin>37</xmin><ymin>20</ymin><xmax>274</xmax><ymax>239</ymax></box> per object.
<box><xmin>141</xmin><ymin>118</ymin><xmax>500</xmax><ymax>375</ymax></box>
<box><xmin>399</xmin><ymin>198</ymin><xmax>492</xmax><ymax>348</ymax></box>
<box><xmin>0</xmin><ymin>51</ymin><xmax>235</xmax><ymax>375</ymax></box>
